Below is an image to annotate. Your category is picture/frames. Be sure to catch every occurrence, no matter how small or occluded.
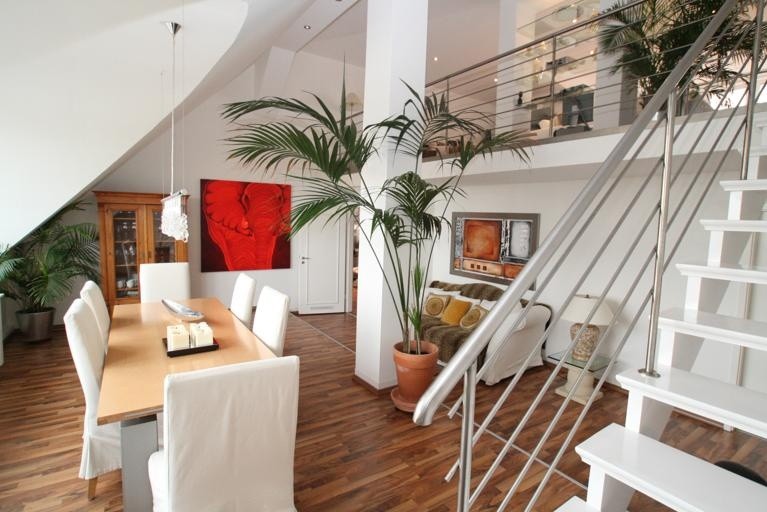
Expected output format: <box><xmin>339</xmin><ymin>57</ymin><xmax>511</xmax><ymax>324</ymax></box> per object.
<box><xmin>450</xmin><ymin>212</ymin><xmax>536</xmax><ymax>292</ymax></box>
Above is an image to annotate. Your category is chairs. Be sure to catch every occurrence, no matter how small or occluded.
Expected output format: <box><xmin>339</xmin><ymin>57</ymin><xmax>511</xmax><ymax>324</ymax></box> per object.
<box><xmin>229</xmin><ymin>271</ymin><xmax>257</xmax><ymax>332</ymax></box>
<box><xmin>138</xmin><ymin>263</ymin><xmax>190</xmax><ymax>305</ymax></box>
<box><xmin>62</xmin><ymin>298</ymin><xmax>162</xmax><ymax>502</ymax></box>
<box><xmin>79</xmin><ymin>279</ymin><xmax>112</xmax><ymax>356</ymax></box>
<box><xmin>142</xmin><ymin>352</ymin><xmax>300</xmax><ymax>512</ymax></box>
<box><xmin>252</xmin><ymin>284</ymin><xmax>291</xmax><ymax>359</ymax></box>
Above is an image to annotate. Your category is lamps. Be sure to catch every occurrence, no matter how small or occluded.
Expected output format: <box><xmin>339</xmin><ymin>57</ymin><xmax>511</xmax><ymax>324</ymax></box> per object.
<box><xmin>562</xmin><ymin>294</ymin><xmax>617</xmax><ymax>361</ymax></box>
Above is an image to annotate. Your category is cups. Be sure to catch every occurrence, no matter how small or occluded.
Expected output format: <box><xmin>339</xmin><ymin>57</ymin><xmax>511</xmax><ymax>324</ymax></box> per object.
<box><xmin>116</xmin><ymin>278</ymin><xmax>138</xmax><ymax>288</ymax></box>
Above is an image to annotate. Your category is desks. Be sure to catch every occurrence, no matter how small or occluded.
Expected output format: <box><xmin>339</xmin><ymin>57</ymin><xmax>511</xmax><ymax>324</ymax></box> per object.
<box><xmin>94</xmin><ymin>298</ymin><xmax>280</xmax><ymax>512</ymax></box>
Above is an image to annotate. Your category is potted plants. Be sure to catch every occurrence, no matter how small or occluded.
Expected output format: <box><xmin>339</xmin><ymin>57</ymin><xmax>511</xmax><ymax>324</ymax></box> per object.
<box><xmin>224</xmin><ymin>56</ymin><xmax>534</xmax><ymax>414</ymax></box>
<box><xmin>0</xmin><ymin>196</ymin><xmax>99</xmax><ymax>342</ymax></box>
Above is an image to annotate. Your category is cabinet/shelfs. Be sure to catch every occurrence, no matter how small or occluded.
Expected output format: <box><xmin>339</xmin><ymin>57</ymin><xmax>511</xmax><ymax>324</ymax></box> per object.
<box><xmin>93</xmin><ymin>191</ymin><xmax>189</xmax><ymax>304</ymax></box>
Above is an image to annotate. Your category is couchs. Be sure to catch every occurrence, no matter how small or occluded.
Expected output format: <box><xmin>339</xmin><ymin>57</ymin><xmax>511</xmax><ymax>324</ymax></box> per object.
<box><xmin>416</xmin><ymin>281</ymin><xmax>550</xmax><ymax>388</ymax></box>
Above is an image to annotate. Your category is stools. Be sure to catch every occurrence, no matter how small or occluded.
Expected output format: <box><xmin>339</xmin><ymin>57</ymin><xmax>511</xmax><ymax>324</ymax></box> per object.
<box><xmin>550</xmin><ymin>351</ymin><xmax>613</xmax><ymax>406</ymax></box>
